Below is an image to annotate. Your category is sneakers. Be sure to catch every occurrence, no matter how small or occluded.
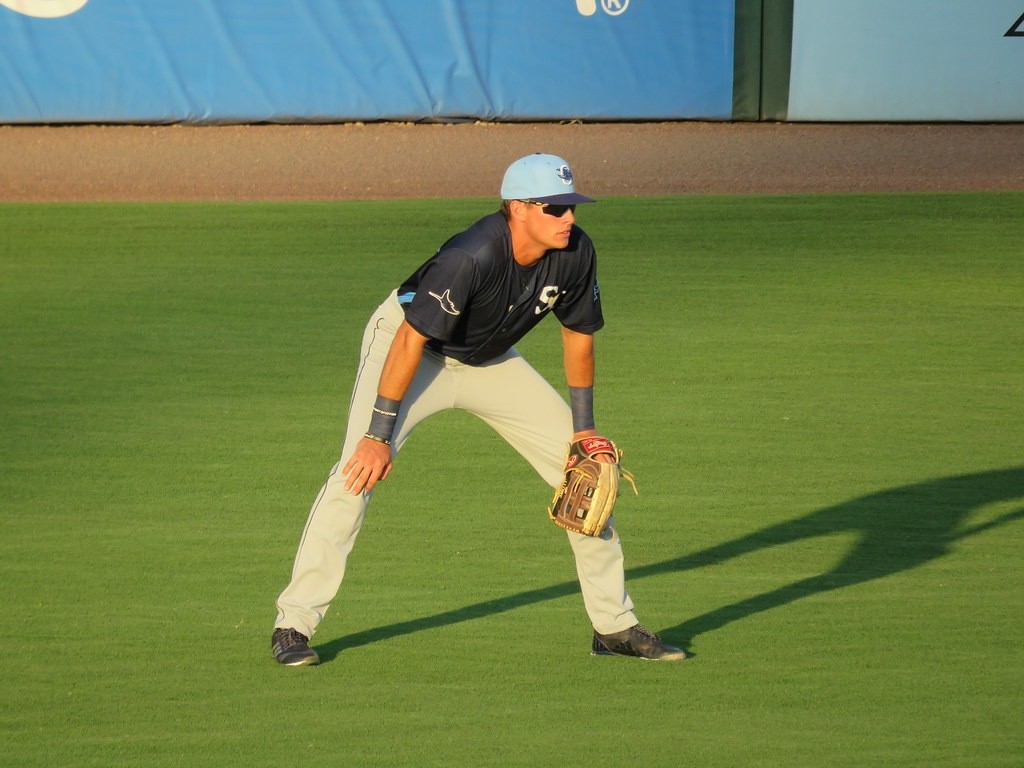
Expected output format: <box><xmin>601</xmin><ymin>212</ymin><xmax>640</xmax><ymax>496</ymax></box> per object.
<box><xmin>272</xmin><ymin>628</ymin><xmax>320</xmax><ymax>666</ymax></box>
<box><xmin>592</xmin><ymin>624</ymin><xmax>686</xmax><ymax>661</ymax></box>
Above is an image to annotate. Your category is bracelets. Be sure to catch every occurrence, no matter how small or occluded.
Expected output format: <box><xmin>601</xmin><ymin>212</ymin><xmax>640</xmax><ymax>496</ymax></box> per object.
<box><xmin>363</xmin><ymin>432</ymin><xmax>391</xmax><ymax>445</ymax></box>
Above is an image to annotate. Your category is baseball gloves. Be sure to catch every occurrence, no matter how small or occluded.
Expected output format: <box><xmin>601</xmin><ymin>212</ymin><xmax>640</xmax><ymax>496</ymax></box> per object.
<box><xmin>545</xmin><ymin>434</ymin><xmax>624</xmax><ymax>538</ymax></box>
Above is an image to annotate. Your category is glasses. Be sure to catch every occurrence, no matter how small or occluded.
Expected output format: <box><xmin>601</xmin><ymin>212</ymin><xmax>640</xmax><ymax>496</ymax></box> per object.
<box><xmin>512</xmin><ymin>199</ymin><xmax>576</xmax><ymax>217</ymax></box>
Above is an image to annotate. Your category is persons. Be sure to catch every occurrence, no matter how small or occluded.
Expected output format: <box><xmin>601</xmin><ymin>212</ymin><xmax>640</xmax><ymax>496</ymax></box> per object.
<box><xmin>270</xmin><ymin>153</ymin><xmax>687</xmax><ymax>671</ymax></box>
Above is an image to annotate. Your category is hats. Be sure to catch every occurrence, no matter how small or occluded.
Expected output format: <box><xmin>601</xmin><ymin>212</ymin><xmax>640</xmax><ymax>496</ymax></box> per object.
<box><xmin>501</xmin><ymin>152</ymin><xmax>597</xmax><ymax>205</ymax></box>
